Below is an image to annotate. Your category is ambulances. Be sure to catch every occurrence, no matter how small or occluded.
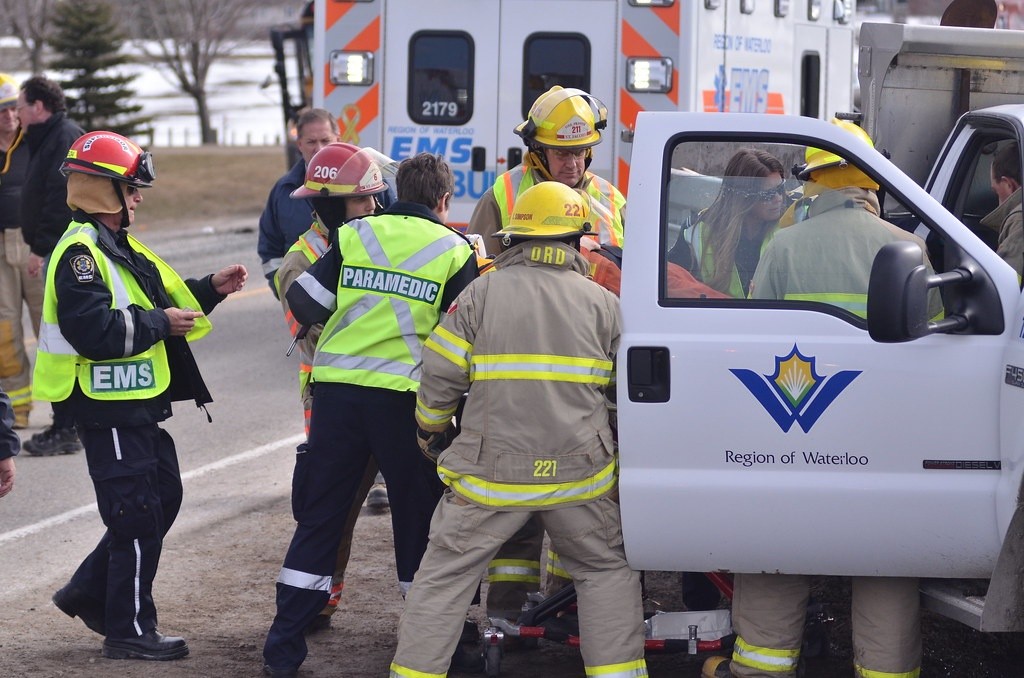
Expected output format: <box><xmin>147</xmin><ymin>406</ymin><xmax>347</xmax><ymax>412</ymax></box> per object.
<box><xmin>261</xmin><ymin>0</ymin><xmax>860</xmax><ymax>239</ymax></box>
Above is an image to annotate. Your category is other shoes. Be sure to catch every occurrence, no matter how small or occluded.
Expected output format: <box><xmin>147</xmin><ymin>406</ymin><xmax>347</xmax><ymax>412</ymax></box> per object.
<box><xmin>263</xmin><ymin>659</ymin><xmax>296</xmax><ymax>678</ymax></box>
<box><xmin>448</xmin><ymin>645</ymin><xmax>484</xmax><ymax>672</ymax></box>
<box><xmin>12</xmin><ymin>410</ymin><xmax>28</xmax><ymax>428</ymax></box>
<box><xmin>301</xmin><ymin>614</ymin><xmax>330</xmax><ymax>635</ymax></box>
<box><xmin>459</xmin><ymin>621</ymin><xmax>480</xmax><ymax>645</ymax></box>
<box><xmin>502</xmin><ymin>619</ymin><xmax>537</xmax><ymax>651</ymax></box>
<box><xmin>700</xmin><ymin>656</ymin><xmax>738</xmax><ymax>678</ymax></box>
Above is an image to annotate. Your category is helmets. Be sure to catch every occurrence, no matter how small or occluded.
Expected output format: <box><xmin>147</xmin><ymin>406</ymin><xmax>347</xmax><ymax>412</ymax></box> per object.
<box><xmin>59</xmin><ymin>131</ymin><xmax>155</xmax><ymax>188</ymax></box>
<box><xmin>513</xmin><ymin>85</ymin><xmax>603</xmax><ymax>150</ymax></box>
<box><xmin>797</xmin><ymin>115</ymin><xmax>876</xmax><ymax>181</ymax></box>
<box><xmin>0</xmin><ymin>74</ymin><xmax>19</xmax><ymax>112</ymax></box>
<box><xmin>289</xmin><ymin>142</ymin><xmax>390</xmax><ymax>200</ymax></box>
<box><xmin>490</xmin><ymin>181</ymin><xmax>598</xmax><ymax>239</ymax></box>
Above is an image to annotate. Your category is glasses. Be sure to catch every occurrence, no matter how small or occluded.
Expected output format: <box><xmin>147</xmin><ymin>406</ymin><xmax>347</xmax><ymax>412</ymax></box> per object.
<box><xmin>549</xmin><ymin>147</ymin><xmax>590</xmax><ymax>161</ymax></box>
<box><xmin>128</xmin><ymin>183</ymin><xmax>138</xmax><ymax>194</ymax></box>
<box><xmin>757</xmin><ymin>179</ymin><xmax>787</xmax><ymax>200</ymax></box>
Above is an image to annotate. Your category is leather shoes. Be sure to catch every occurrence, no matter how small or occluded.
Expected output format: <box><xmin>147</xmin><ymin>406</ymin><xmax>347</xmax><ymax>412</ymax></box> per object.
<box><xmin>53</xmin><ymin>582</ymin><xmax>105</xmax><ymax>635</ymax></box>
<box><xmin>102</xmin><ymin>628</ymin><xmax>189</xmax><ymax>660</ymax></box>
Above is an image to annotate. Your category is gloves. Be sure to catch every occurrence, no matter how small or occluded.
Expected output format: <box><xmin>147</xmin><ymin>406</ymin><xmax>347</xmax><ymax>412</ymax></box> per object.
<box><xmin>416</xmin><ymin>427</ymin><xmax>454</xmax><ymax>465</ymax></box>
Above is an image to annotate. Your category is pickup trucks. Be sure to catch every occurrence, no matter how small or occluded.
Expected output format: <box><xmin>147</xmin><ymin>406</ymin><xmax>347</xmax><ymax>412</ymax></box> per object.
<box><xmin>613</xmin><ymin>0</ymin><xmax>1024</xmax><ymax>636</ymax></box>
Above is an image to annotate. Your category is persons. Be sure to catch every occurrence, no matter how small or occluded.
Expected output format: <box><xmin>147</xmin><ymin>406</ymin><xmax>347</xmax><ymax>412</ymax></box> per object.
<box><xmin>30</xmin><ymin>130</ymin><xmax>249</xmax><ymax>661</ymax></box>
<box><xmin>263</xmin><ymin>151</ymin><xmax>486</xmax><ymax>678</ymax></box>
<box><xmin>257</xmin><ymin>109</ymin><xmax>398</xmax><ymax>508</ymax></box>
<box><xmin>273</xmin><ymin>142</ymin><xmax>400</xmax><ymax>640</ymax></box>
<box><xmin>389</xmin><ymin>181</ymin><xmax>649</xmax><ymax>678</ymax></box>
<box><xmin>464</xmin><ymin>85</ymin><xmax>627</xmax><ymax>652</ymax></box>
<box><xmin>465</xmin><ymin>233</ymin><xmax>736</xmax><ymax>299</ymax></box>
<box><xmin>666</xmin><ymin>149</ymin><xmax>813</xmax><ymax>612</ymax></box>
<box><xmin>701</xmin><ymin>117</ymin><xmax>945</xmax><ymax>678</ymax></box>
<box><xmin>0</xmin><ymin>73</ymin><xmax>45</xmax><ymax>427</ymax></box>
<box><xmin>979</xmin><ymin>139</ymin><xmax>1024</xmax><ymax>286</ymax></box>
<box><xmin>15</xmin><ymin>77</ymin><xmax>87</xmax><ymax>456</ymax></box>
<box><xmin>0</xmin><ymin>386</ymin><xmax>20</xmax><ymax>498</ymax></box>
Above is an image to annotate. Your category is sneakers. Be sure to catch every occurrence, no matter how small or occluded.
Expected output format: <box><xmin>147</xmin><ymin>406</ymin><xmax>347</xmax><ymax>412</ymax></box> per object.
<box><xmin>22</xmin><ymin>423</ymin><xmax>83</xmax><ymax>455</ymax></box>
<box><xmin>367</xmin><ymin>483</ymin><xmax>389</xmax><ymax>508</ymax></box>
